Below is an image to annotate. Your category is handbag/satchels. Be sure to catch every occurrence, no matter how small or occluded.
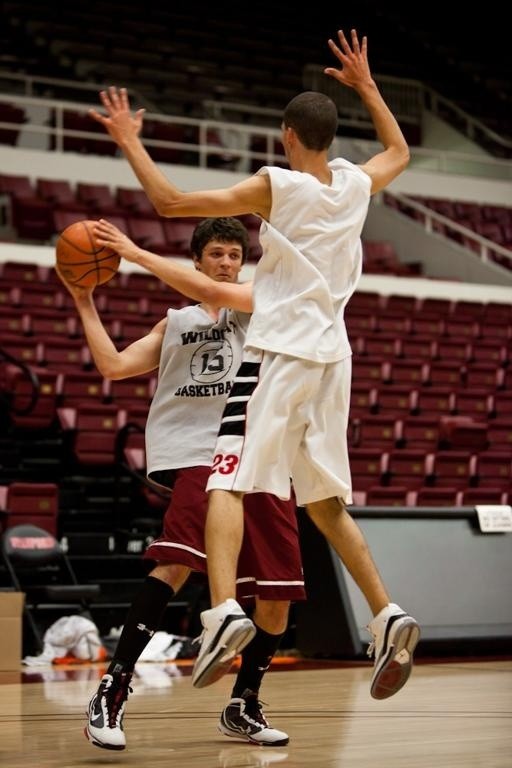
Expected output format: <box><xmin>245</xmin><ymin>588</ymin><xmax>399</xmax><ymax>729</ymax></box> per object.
<box><xmin>71</xmin><ymin>632</ymin><xmax>106</xmax><ymax>663</ymax></box>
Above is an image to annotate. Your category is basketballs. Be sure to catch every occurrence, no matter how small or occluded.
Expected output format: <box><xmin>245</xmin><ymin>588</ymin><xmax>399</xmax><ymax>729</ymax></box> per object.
<box><xmin>56</xmin><ymin>220</ymin><xmax>120</xmax><ymax>287</ymax></box>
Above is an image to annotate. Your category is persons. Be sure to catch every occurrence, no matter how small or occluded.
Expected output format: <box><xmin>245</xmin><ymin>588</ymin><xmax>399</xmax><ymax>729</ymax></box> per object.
<box><xmin>47</xmin><ymin>211</ymin><xmax>309</xmax><ymax>753</ymax></box>
<box><xmin>81</xmin><ymin>20</ymin><xmax>422</xmax><ymax>703</ymax></box>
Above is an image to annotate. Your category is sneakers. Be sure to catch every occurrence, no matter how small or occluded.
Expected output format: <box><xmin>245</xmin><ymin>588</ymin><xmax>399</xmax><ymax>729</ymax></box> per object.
<box><xmin>217</xmin><ymin>697</ymin><xmax>289</xmax><ymax>745</ymax></box>
<box><xmin>84</xmin><ymin>673</ymin><xmax>128</xmax><ymax>748</ymax></box>
<box><xmin>191</xmin><ymin>598</ymin><xmax>257</xmax><ymax>687</ymax></box>
<box><xmin>365</xmin><ymin>602</ymin><xmax>421</xmax><ymax>698</ymax></box>
